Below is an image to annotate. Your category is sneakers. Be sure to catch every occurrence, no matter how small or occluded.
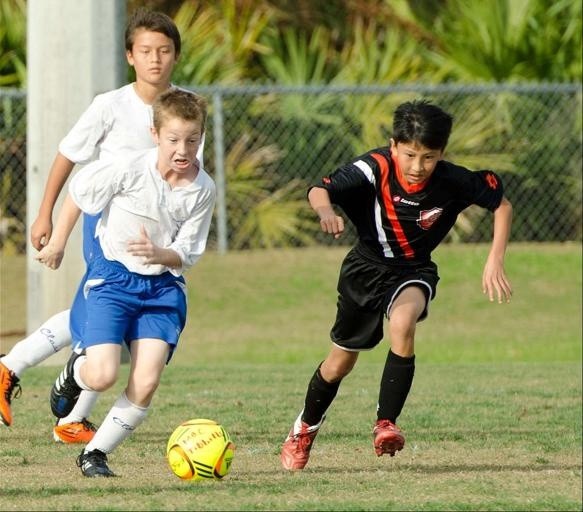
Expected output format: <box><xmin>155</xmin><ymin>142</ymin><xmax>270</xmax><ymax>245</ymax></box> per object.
<box><xmin>0</xmin><ymin>351</ymin><xmax>21</xmax><ymax>429</ymax></box>
<box><xmin>53</xmin><ymin>422</ymin><xmax>102</xmax><ymax>444</ymax></box>
<box><xmin>76</xmin><ymin>449</ymin><xmax>117</xmax><ymax>480</ymax></box>
<box><xmin>282</xmin><ymin>409</ymin><xmax>326</xmax><ymax>473</ymax></box>
<box><xmin>371</xmin><ymin>418</ymin><xmax>405</xmax><ymax>457</ymax></box>
<box><xmin>50</xmin><ymin>347</ymin><xmax>88</xmax><ymax>418</ymax></box>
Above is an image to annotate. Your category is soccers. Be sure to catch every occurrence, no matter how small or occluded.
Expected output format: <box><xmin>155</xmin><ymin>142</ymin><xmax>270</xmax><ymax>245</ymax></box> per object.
<box><xmin>166</xmin><ymin>418</ymin><xmax>235</xmax><ymax>481</ymax></box>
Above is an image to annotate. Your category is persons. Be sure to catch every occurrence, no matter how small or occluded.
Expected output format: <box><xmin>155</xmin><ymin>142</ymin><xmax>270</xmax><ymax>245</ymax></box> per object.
<box><xmin>279</xmin><ymin>101</ymin><xmax>514</xmax><ymax>472</ymax></box>
<box><xmin>34</xmin><ymin>90</ymin><xmax>218</xmax><ymax>479</ymax></box>
<box><xmin>1</xmin><ymin>8</ymin><xmax>206</xmax><ymax>445</ymax></box>
